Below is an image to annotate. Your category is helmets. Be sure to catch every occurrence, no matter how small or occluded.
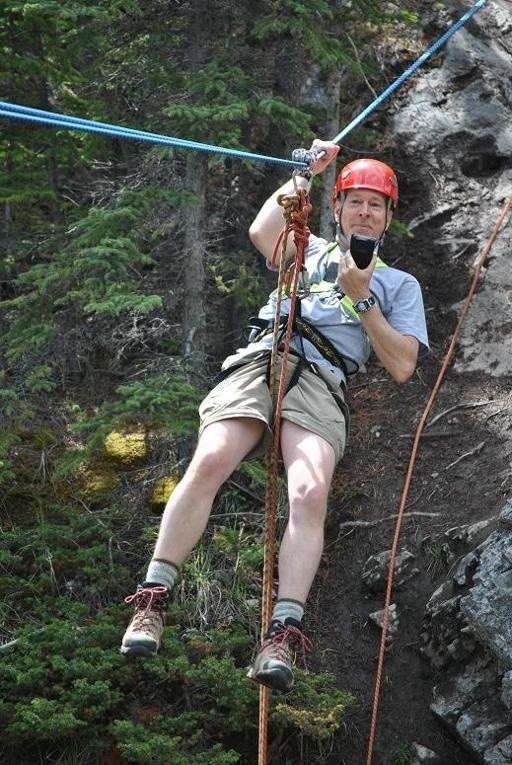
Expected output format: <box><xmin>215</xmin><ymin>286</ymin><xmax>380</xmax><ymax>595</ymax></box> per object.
<box><xmin>333</xmin><ymin>158</ymin><xmax>400</xmax><ymax>209</ymax></box>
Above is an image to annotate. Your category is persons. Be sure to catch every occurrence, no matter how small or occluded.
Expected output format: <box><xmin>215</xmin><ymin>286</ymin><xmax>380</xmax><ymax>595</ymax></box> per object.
<box><xmin>120</xmin><ymin>138</ymin><xmax>434</xmax><ymax>696</ymax></box>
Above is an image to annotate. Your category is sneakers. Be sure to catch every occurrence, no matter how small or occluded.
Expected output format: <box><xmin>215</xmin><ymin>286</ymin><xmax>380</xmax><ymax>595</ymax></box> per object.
<box><xmin>246</xmin><ymin>616</ymin><xmax>313</xmax><ymax>691</ymax></box>
<box><xmin>121</xmin><ymin>582</ymin><xmax>170</xmax><ymax>655</ymax></box>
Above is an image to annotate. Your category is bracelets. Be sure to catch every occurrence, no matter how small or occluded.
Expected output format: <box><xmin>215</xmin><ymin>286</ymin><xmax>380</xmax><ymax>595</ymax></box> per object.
<box><xmin>298</xmin><ymin>171</ymin><xmax>314</xmax><ymax>183</ymax></box>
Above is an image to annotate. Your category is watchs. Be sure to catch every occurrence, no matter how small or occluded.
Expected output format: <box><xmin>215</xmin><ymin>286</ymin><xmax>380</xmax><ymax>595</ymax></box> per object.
<box><xmin>352</xmin><ymin>295</ymin><xmax>376</xmax><ymax>314</ymax></box>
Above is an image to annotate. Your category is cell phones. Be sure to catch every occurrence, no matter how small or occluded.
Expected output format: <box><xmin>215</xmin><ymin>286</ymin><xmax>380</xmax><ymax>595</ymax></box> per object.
<box><xmin>350</xmin><ymin>233</ymin><xmax>376</xmax><ymax>269</ymax></box>
